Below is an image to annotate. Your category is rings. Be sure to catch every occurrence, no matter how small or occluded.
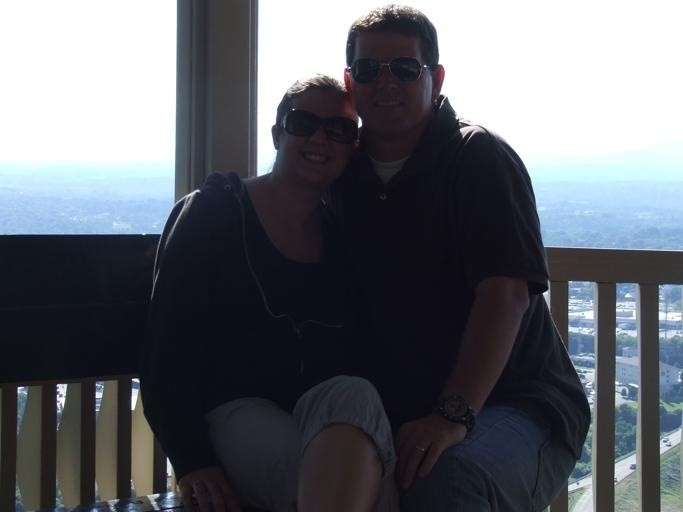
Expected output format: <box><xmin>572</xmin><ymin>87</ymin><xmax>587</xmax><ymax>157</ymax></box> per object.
<box><xmin>415</xmin><ymin>443</ymin><xmax>426</xmax><ymax>453</ymax></box>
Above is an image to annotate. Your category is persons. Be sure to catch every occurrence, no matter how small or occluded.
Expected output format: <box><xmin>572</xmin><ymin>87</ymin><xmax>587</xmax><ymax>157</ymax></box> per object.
<box><xmin>320</xmin><ymin>6</ymin><xmax>591</xmax><ymax>512</ymax></box>
<box><xmin>138</xmin><ymin>77</ymin><xmax>397</xmax><ymax>511</ymax></box>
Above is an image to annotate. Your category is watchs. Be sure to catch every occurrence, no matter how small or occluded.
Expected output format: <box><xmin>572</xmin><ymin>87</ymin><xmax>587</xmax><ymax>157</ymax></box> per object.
<box><xmin>431</xmin><ymin>391</ymin><xmax>477</xmax><ymax>440</ymax></box>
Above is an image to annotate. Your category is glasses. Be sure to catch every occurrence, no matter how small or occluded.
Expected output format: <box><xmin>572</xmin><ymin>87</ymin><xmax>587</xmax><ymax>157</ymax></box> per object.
<box><xmin>347</xmin><ymin>56</ymin><xmax>431</xmax><ymax>84</ymax></box>
<box><xmin>281</xmin><ymin>109</ymin><xmax>359</xmax><ymax>144</ymax></box>
